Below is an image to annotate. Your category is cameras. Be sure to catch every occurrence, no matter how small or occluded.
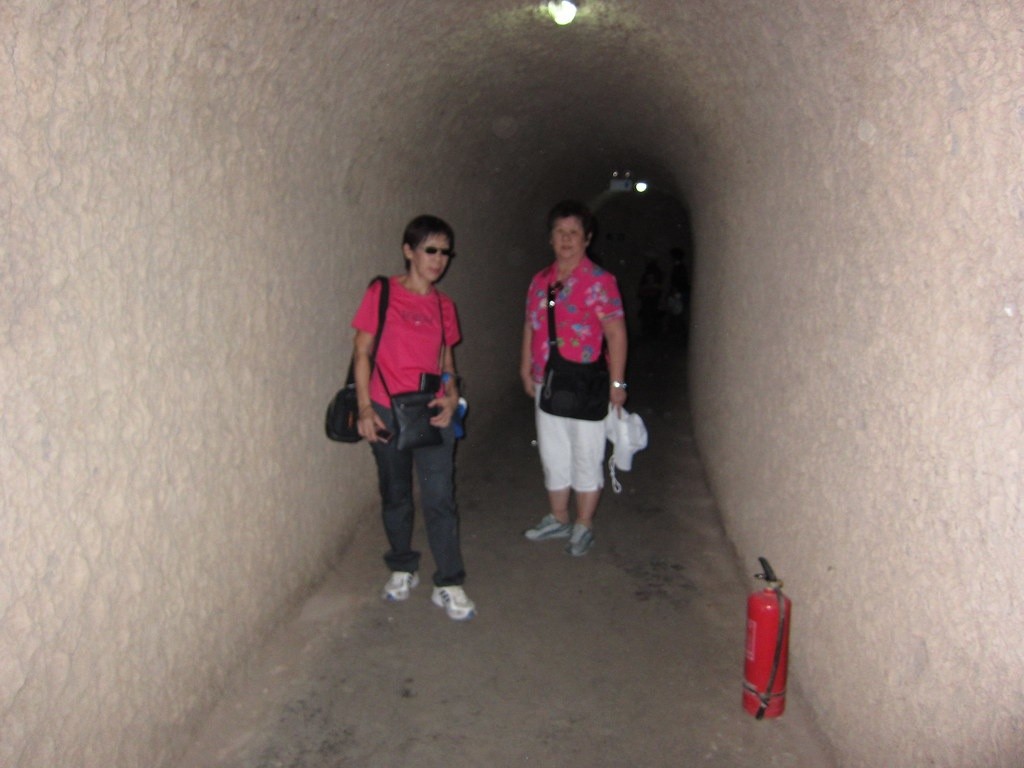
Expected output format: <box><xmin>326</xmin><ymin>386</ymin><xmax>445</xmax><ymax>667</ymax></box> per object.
<box><xmin>375</xmin><ymin>426</ymin><xmax>394</xmax><ymax>445</ymax></box>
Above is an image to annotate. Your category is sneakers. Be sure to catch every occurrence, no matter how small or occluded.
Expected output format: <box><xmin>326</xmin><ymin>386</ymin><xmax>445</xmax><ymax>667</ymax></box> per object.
<box><xmin>523</xmin><ymin>511</ymin><xmax>572</xmax><ymax>540</ymax></box>
<box><xmin>380</xmin><ymin>570</ymin><xmax>420</xmax><ymax>600</ymax></box>
<box><xmin>562</xmin><ymin>524</ymin><xmax>596</xmax><ymax>557</ymax></box>
<box><xmin>430</xmin><ymin>585</ymin><xmax>478</xmax><ymax>622</ymax></box>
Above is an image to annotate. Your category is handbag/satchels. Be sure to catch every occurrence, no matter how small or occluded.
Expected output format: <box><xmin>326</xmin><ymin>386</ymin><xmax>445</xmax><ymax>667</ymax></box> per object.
<box><xmin>325</xmin><ymin>386</ymin><xmax>363</xmax><ymax>443</ymax></box>
<box><xmin>539</xmin><ymin>341</ymin><xmax>611</xmax><ymax>420</ymax></box>
<box><xmin>390</xmin><ymin>391</ymin><xmax>443</xmax><ymax>453</ymax></box>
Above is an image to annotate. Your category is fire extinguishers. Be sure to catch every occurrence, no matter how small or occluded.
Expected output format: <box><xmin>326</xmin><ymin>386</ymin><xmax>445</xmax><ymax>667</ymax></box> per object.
<box><xmin>743</xmin><ymin>557</ymin><xmax>792</xmax><ymax>720</ymax></box>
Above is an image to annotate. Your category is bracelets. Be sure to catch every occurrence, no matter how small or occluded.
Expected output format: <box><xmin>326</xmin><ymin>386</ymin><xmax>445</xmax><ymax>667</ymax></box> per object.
<box><xmin>358</xmin><ymin>403</ymin><xmax>371</xmax><ymax>415</ymax></box>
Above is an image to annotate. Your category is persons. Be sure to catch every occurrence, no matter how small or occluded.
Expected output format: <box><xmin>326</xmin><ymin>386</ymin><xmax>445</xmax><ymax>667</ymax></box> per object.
<box><xmin>351</xmin><ymin>215</ymin><xmax>480</xmax><ymax>620</ymax></box>
<box><xmin>520</xmin><ymin>201</ymin><xmax>628</xmax><ymax>557</ymax></box>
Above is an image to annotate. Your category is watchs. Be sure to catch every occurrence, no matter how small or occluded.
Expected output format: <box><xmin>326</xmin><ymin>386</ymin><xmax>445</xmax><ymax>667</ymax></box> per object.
<box><xmin>609</xmin><ymin>382</ymin><xmax>625</xmax><ymax>388</ymax></box>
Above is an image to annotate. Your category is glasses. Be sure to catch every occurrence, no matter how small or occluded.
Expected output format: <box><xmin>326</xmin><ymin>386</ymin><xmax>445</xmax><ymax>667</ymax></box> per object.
<box><xmin>416</xmin><ymin>244</ymin><xmax>451</xmax><ymax>256</ymax></box>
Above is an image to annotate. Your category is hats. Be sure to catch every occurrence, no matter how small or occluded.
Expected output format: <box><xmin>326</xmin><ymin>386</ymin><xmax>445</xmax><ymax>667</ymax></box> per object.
<box><xmin>606</xmin><ymin>402</ymin><xmax>648</xmax><ymax>472</ymax></box>
